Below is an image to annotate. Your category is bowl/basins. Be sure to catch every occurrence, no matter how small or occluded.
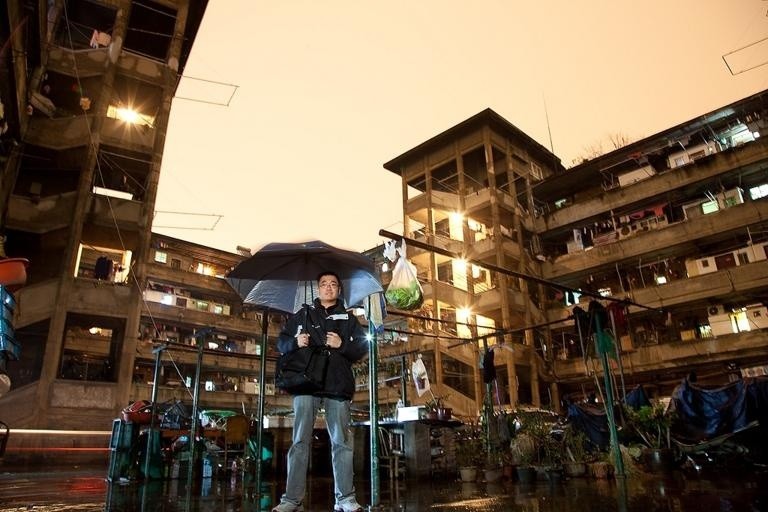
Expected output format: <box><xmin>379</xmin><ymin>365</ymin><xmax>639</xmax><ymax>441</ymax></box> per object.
<box><xmin>1</xmin><ymin>257</ymin><xmax>29</xmax><ymax>290</ymax></box>
<box><xmin>121</xmin><ymin>411</ymin><xmax>152</xmax><ymax>424</ymax></box>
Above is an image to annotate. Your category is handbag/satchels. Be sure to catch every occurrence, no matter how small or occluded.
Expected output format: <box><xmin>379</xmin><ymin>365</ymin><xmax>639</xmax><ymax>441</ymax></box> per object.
<box><xmin>275</xmin><ymin>346</ymin><xmax>330</xmax><ymax>394</ymax></box>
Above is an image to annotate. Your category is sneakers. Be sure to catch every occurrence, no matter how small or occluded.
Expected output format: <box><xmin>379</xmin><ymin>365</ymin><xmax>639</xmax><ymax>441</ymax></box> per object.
<box><xmin>272</xmin><ymin>499</ymin><xmax>304</xmax><ymax>512</ymax></box>
<box><xmin>334</xmin><ymin>496</ymin><xmax>364</xmax><ymax>512</ymax></box>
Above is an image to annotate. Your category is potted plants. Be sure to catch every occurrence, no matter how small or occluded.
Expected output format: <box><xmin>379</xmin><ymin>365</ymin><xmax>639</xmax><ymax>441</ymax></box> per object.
<box><xmin>624</xmin><ymin>399</ymin><xmax>677</xmax><ymax>479</ymax></box>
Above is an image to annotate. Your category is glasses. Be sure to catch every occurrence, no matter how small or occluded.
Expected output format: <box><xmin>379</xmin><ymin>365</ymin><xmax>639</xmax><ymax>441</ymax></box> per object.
<box><xmin>319</xmin><ymin>282</ymin><xmax>340</xmax><ymax>289</ymax></box>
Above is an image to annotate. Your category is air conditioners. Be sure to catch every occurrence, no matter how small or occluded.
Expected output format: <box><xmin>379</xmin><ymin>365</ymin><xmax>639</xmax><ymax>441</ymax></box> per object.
<box><xmin>707</xmin><ymin>303</ymin><xmax>726</xmax><ymax>316</ymax></box>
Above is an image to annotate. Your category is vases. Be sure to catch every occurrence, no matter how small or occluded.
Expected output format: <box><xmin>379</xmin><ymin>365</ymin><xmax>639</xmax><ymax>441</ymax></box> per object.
<box><xmin>460</xmin><ymin>466</ymin><xmax>476</xmax><ymax>480</ymax></box>
<box><xmin>426</xmin><ymin>406</ymin><xmax>451</xmax><ymax>419</ymax></box>
<box><xmin>482</xmin><ymin>429</ymin><xmax>615</xmax><ymax>481</ymax></box>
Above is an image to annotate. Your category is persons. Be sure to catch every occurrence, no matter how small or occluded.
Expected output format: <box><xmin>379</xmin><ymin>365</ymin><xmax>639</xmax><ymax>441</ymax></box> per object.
<box><xmin>268</xmin><ymin>270</ymin><xmax>370</xmax><ymax>512</ymax></box>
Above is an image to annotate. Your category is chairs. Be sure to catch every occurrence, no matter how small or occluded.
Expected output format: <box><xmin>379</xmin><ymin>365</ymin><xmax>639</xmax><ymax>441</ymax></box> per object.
<box><xmin>205</xmin><ymin>414</ymin><xmax>249</xmax><ymax>474</ymax></box>
<box><xmin>378</xmin><ymin>427</ymin><xmax>406</xmax><ymax>481</ymax></box>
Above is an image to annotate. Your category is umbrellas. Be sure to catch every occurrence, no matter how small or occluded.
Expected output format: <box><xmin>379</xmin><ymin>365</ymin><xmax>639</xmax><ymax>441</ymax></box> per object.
<box><xmin>225</xmin><ymin>239</ymin><xmax>385</xmax><ymax>339</ymax></box>
